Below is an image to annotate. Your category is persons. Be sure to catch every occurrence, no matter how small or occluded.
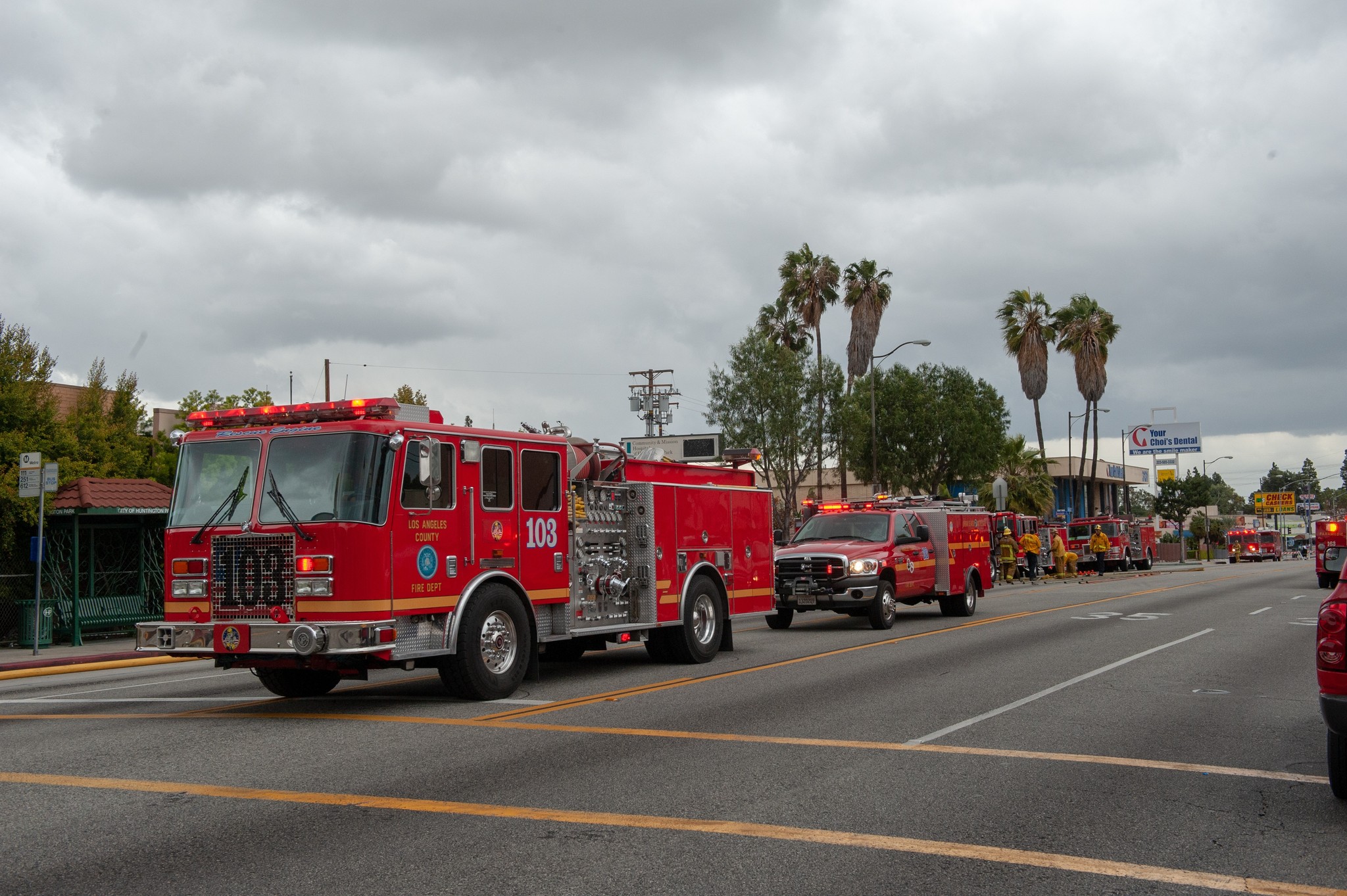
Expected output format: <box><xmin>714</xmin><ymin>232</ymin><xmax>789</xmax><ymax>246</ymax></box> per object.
<box><xmin>1046</xmin><ymin>528</ymin><xmax>1066</xmax><ymax>579</ymax></box>
<box><xmin>1090</xmin><ymin>525</ymin><xmax>1110</xmax><ymax>576</ymax></box>
<box><xmin>1062</xmin><ymin>552</ymin><xmax>1078</xmax><ymax>579</ymax></box>
<box><xmin>1275</xmin><ymin>549</ymin><xmax>1281</xmax><ymax>561</ymax></box>
<box><xmin>1019</xmin><ymin>529</ymin><xmax>1041</xmax><ymax>581</ymax></box>
<box><xmin>1232</xmin><ymin>540</ymin><xmax>1241</xmax><ymax>563</ymax></box>
<box><xmin>997</xmin><ymin>528</ymin><xmax>1019</xmax><ymax>583</ymax></box>
<box><xmin>1302</xmin><ymin>543</ymin><xmax>1307</xmax><ymax>560</ymax></box>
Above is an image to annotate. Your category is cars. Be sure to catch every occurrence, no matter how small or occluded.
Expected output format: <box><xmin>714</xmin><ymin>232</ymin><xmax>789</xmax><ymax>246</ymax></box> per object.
<box><xmin>1316</xmin><ymin>556</ymin><xmax>1347</xmax><ymax>803</ymax></box>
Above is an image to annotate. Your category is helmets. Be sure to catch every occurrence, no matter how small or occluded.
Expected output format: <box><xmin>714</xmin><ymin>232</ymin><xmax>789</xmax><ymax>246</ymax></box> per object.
<box><xmin>1050</xmin><ymin>529</ymin><xmax>1059</xmax><ymax>534</ymax></box>
<box><xmin>1095</xmin><ymin>525</ymin><xmax>1101</xmax><ymax>530</ymax></box>
<box><xmin>1003</xmin><ymin>528</ymin><xmax>1012</xmax><ymax>535</ymax></box>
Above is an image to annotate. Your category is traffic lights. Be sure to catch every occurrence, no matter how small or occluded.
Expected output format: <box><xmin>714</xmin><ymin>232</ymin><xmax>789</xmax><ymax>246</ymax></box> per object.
<box><xmin>1288</xmin><ymin>527</ymin><xmax>1291</xmax><ymax>534</ymax></box>
<box><xmin>1297</xmin><ymin>505</ymin><xmax>1306</xmax><ymax>516</ymax></box>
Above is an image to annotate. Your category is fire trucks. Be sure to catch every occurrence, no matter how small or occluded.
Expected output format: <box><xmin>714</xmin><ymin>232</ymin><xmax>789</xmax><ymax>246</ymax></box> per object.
<box><xmin>134</xmin><ymin>397</ymin><xmax>779</xmax><ymax>701</ymax></box>
<box><xmin>987</xmin><ymin>511</ymin><xmax>1069</xmax><ymax>583</ymax></box>
<box><xmin>1224</xmin><ymin>527</ymin><xmax>1282</xmax><ymax>564</ymax></box>
<box><xmin>1315</xmin><ymin>521</ymin><xmax>1347</xmax><ymax>589</ymax></box>
<box><xmin>764</xmin><ymin>493</ymin><xmax>994</xmax><ymax>631</ymax></box>
<box><xmin>1066</xmin><ymin>511</ymin><xmax>1158</xmax><ymax>572</ymax></box>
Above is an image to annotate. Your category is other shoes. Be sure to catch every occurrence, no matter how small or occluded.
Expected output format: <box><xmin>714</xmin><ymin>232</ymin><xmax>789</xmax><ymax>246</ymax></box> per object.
<box><xmin>1099</xmin><ymin>573</ymin><xmax>1103</xmax><ymax>576</ymax></box>
<box><xmin>1071</xmin><ymin>575</ymin><xmax>1077</xmax><ymax>577</ymax></box>
<box><xmin>1006</xmin><ymin>580</ymin><xmax>1013</xmax><ymax>583</ymax></box>
<box><xmin>1030</xmin><ymin>579</ymin><xmax>1037</xmax><ymax>581</ymax></box>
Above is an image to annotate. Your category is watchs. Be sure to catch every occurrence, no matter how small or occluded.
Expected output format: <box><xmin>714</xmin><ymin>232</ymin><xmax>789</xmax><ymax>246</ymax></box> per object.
<box><xmin>1090</xmin><ymin>550</ymin><xmax>1092</xmax><ymax>551</ymax></box>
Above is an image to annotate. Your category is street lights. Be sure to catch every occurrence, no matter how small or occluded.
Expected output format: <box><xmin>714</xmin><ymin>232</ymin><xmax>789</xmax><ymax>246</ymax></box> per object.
<box><xmin>1260</xmin><ymin>474</ymin><xmax>1283</xmax><ymax>527</ymax></box>
<box><xmin>1122</xmin><ymin>423</ymin><xmax>1153</xmax><ymax>514</ymax></box>
<box><xmin>1203</xmin><ymin>455</ymin><xmax>1234</xmax><ymax>562</ymax></box>
<box><xmin>871</xmin><ymin>339</ymin><xmax>932</xmax><ymax>497</ymax></box>
<box><xmin>1298</xmin><ymin>487</ymin><xmax>1308</xmax><ymax>533</ymax></box>
<box><xmin>1069</xmin><ymin>408</ymin><xmax>1110</xmax><ymax>523</ymax></box>
<box><xmin>1283</xmin><ymin>487</ymin><xmax>1286</xmax><ymax>537</ymax></box>
<box><xmin>1279</xmin><ymin>477</ymin><xmax>1321</xmax><ymax>553</ymax></box>
